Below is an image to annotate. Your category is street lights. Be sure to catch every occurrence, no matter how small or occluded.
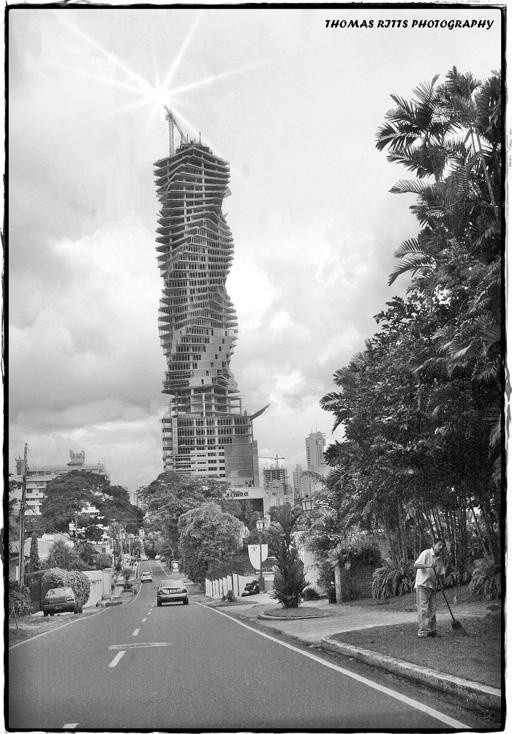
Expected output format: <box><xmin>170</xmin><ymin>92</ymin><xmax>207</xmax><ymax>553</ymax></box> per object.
<box><xmin>300</xmin><ymin>494</ymin><xmax>314</xmax><ymax>533</ymax></box>
<box><xmin>255</xmin><ymin>515</ymin><xmax>265</xmax><ymax>594</ymax></box>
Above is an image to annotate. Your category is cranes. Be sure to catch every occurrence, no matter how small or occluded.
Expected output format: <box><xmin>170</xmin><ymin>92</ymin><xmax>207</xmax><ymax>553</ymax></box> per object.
<box><xmin>257</xmin><ymin>454</ymin><xmax>286</xmax><ymax>468</ymax></box>
<box><xmin>161</xmin><ymin>105</ymin><xmax>187</xmax><ymax>155</ymax></box>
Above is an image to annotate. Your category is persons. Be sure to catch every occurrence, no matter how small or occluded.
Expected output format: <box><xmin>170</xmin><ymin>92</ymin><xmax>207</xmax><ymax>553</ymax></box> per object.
<box><xmin>411</xmin><ymin>535</ymin><xmax>449</xmax><ymax>638</ymax></box>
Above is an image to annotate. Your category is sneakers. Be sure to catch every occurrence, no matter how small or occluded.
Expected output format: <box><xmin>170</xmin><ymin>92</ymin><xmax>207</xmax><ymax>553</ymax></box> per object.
<box><xmin>417</xmin><ymin>630</ymin><xmax>441</xmax><ymax>638</ymax></box>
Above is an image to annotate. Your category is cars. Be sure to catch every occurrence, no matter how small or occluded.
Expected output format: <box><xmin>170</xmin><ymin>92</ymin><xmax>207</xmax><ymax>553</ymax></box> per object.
<box><xmin>157</xmin><ymin>580</ymin><xmax>188</xmax><ymax>606</ymax></box>
<box><xmin>42</xmin><ymin>587</ymin><xmax>83</xmax><ymax>617</ymax></box>
<box><xmin>140</xmin><ymin>572</ymin><xmax>153</xmax><ymax>583</ymax></box>
<box><xmin>142</xmin><ymin>555</ymin><xmax>167</xmax><ymax>562</ymax></box>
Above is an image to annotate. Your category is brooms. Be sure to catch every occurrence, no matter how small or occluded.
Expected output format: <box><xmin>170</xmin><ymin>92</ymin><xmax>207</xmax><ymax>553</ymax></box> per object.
<box><xmin>433</xmin><ymin>567</ymin><xmax>468</xmax><ymax>637</ymax></box>
